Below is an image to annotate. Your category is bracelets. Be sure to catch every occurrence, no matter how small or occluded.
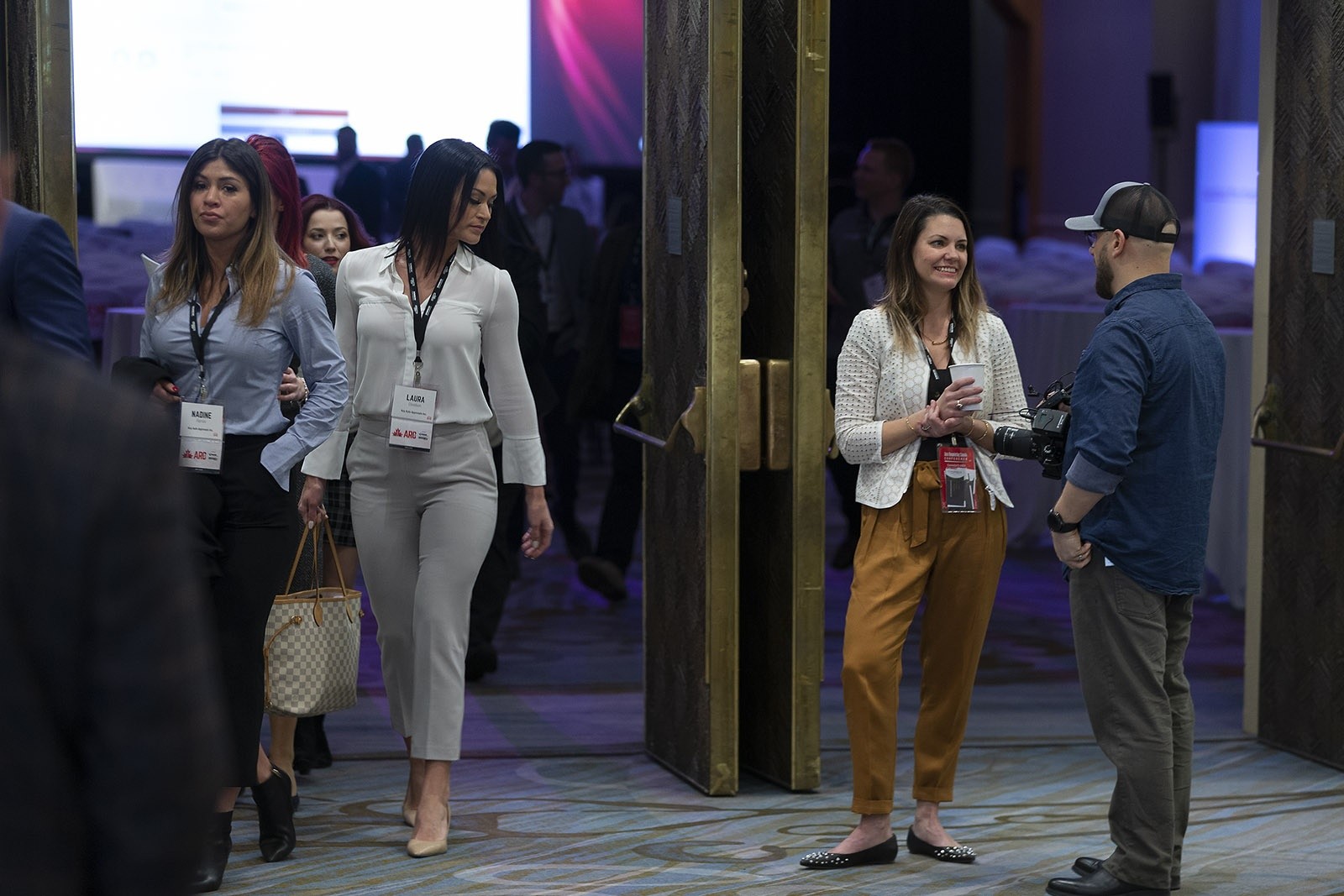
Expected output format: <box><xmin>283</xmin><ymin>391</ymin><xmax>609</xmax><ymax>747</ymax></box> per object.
<box><xmin>905</xmin><ymin>415</ymin><xmax>915</xmax><ymax>430</ymax></box>
<box><xmin>291</xmin><ymin>376</ymin><xmax>309</xmax><ymax>405</ymax></box>
<box><xmin>976</xmin><ymin>420</ymin><xmax>988</xmax><ymax>443</ymax></box>
<box><xmin>958</xmin><ymin>417</ymin><xmax>975</xmax><ymax>437</ymax></box>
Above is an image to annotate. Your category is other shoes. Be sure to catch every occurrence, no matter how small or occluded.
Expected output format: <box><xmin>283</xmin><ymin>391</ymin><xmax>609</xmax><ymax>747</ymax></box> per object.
<box><xmin>800</xmin><ymin>834</ymin><xmax>898</xmax><ymax>867</ymax></box>
<box><xmin>906</xmin><ymin>825</ymin><xmax>977</xmax><ymax>864</ymax></box>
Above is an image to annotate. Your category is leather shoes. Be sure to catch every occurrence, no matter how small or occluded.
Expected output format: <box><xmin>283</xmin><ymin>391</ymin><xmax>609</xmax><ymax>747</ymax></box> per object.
<box><xmin>1045</xmin><ymin>869</ymin><xmax>1174</xmax><ymax>896</ymax></box>
<box><xmin>1072</xmin><ymin>857</ymin><xmax>1182</xmax><ymax>890</ymax></box>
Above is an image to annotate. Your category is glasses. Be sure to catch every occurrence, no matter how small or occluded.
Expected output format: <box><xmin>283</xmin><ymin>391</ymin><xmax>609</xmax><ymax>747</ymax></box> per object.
<box><xmin>1084</xmin><ymin>229</ymin><xmax>1129</xmax><ymax>249</ymax></box>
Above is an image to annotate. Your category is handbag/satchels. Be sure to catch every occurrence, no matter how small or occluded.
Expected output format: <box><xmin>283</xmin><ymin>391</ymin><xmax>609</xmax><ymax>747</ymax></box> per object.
<box><xmin>264</xmin><ymin>512</ymin><xmax>365</xmax><ymax>717</ymax></box>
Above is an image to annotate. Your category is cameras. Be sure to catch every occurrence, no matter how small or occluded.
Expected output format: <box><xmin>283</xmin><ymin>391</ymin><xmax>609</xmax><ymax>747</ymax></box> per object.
<box><xmin>993</xmin><ymin>408</ymin><xmax>1071</xmax><ymax>479</ymax></box>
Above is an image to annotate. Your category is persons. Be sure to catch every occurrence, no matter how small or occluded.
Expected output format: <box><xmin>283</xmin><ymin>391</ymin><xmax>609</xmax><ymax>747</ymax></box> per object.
<box><xmin>0</xmin><ymin>120</ymin><xmax>635</xmax><ymax>896</ymax></box>
<box><xmin>1045</xmin><ymin>180</ymin><xmax>1229</xmax><ymax>896</ymax></box>
<box><xmin>799</xmin><ymin>194</ymin><xmax>1031</xmax><ymax>871</ymax></box>
<box><xmin>827</xmin><ymin>136</ymin><xmax>920</xmax><ymax>574</ymax></box>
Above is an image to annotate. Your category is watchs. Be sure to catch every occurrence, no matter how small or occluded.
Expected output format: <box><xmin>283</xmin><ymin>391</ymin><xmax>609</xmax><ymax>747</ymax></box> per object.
<box><xmin>1047</xmin><ymin>508</ymin><xmax>1077</xmax><ymax>532</ymax></box>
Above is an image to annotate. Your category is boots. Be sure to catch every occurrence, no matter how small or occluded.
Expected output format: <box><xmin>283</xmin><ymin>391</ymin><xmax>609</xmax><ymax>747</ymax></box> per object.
<box><xmin>250</xmin><ymin>762</ymin><xmax>297</xmax><ymax>862</ymax></box>
<box><xmin>187</xmin><ymin>810</ymin><xmax>233</xmax><ymax>893</ymax></box>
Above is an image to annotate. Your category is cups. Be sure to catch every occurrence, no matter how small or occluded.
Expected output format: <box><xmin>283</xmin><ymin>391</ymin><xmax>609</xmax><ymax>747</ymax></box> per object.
<box><xmin>948</xmin><ymin>364</ymin><xmax>986</xmax><ymax>411</ymax></box>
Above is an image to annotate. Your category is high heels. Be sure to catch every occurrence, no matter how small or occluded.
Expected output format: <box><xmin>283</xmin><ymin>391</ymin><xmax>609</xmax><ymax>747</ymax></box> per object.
<box><xmin>402</xmin><ymin>809</ymin><xmax>416</xmax><ymax>827</ymax></box>
<box><xmin>407</xmin><ymin>801</ymin><xmax>451</xmax><ymax>857</ymax></box>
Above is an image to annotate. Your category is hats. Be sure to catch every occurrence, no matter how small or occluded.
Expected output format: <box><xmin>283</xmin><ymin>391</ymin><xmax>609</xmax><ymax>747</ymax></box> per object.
<box><xmin>1064</xmin><ymin>181</ymin><xmax>1182</xmax><ymax>243</ymax></box>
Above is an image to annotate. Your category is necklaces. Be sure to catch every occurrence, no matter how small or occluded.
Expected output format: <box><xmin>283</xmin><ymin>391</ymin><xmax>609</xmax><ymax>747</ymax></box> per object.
<box><xmin>920</xmin><ymin>329</ymin><xmax>949</xmax><ymax>345</ymax></box>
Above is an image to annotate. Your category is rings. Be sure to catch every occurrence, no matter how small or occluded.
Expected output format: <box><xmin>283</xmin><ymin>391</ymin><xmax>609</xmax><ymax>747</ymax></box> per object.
<box><xmin>922</xmin><ymin>426</ymin><xmax>931</xmax><ymax>431</ymax></box>
<box><xmin>1079</xmin><ymin>555</ymin><xmax>1082</xmax><ymax>562</ymax></box>
